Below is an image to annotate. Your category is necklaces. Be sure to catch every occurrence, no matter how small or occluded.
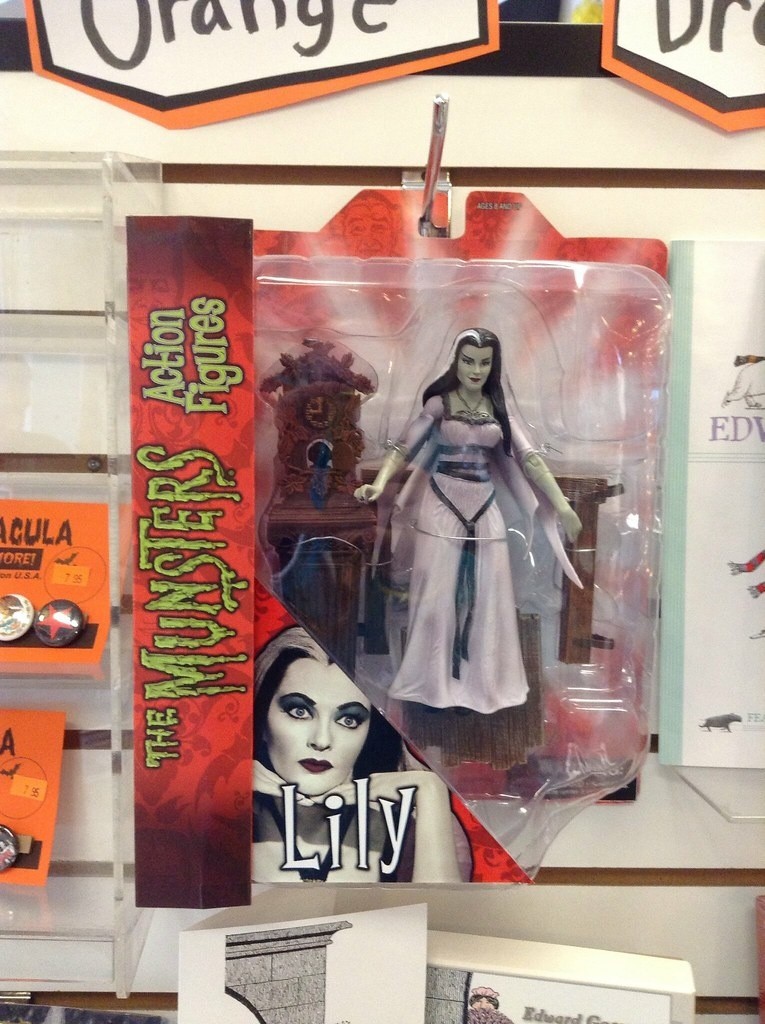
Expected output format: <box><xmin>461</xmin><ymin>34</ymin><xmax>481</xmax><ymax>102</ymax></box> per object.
<box><xmin>455</xmin><ymin>388</ymin><xmax>483</xmax><ymax>412</ymax></box>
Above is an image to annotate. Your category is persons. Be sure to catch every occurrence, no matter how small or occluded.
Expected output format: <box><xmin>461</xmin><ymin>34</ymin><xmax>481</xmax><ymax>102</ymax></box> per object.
<box><xmin>212</xmin><ymin>625</ymin><xmax>472</xmax><ymax>883</ymax></box>
<box><xmin>353</xmin><ymin>329</ymin><xmax>581</xmax><ymax>717</ymax></box>
